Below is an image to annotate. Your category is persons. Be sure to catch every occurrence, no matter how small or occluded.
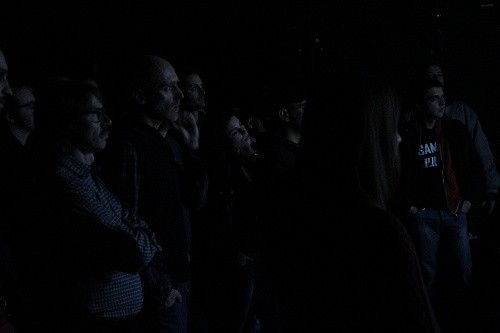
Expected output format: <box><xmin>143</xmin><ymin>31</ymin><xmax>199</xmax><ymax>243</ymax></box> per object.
<box><xmin>398</xmin><ymin>79</ymin><xmax>488</xmax><ymax>306</ymax></box>
<box><xmin>0</xmin><ymin>78</ymin><xmax>35</xmax><ymax>333</ymax></box>
<box><xmin>33</xmin><ymin>83</ymin><xmax>164</xmax><ymax>333</ymax></box>
<box><xmin>170</xmin><ymin>70</ymin><xmax>307</xmax><ymax>331</ymax></box>
<box><xmin>0</xmin><ymin>48</ymin><xmax>17</xmax><ymax>333</ymax></box>
<box><xmin>276</xmin><ymin>70</ymin><xmax>439</xmax><ymax>333</ymax></box>
<box><xmin>400</xmin><ymin>61</ymin><xmax>500</xmax><ymax>214</ymax></box>
<box><xmin>117</xmin><ymin>55</ymin><xmax>207</xmax><ymax>332</ymax></box>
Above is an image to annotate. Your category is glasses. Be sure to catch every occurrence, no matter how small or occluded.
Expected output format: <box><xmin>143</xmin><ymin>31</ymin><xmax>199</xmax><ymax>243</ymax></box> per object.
<box><xmin>13</xmin><ymin>101</ymin><xmax>33</xmax><ymax>110</ymax></box>
<box><xmin>81</xmin><ymin>108</ymin><xmax>105</xmax><ymax>121</ymax></box>
